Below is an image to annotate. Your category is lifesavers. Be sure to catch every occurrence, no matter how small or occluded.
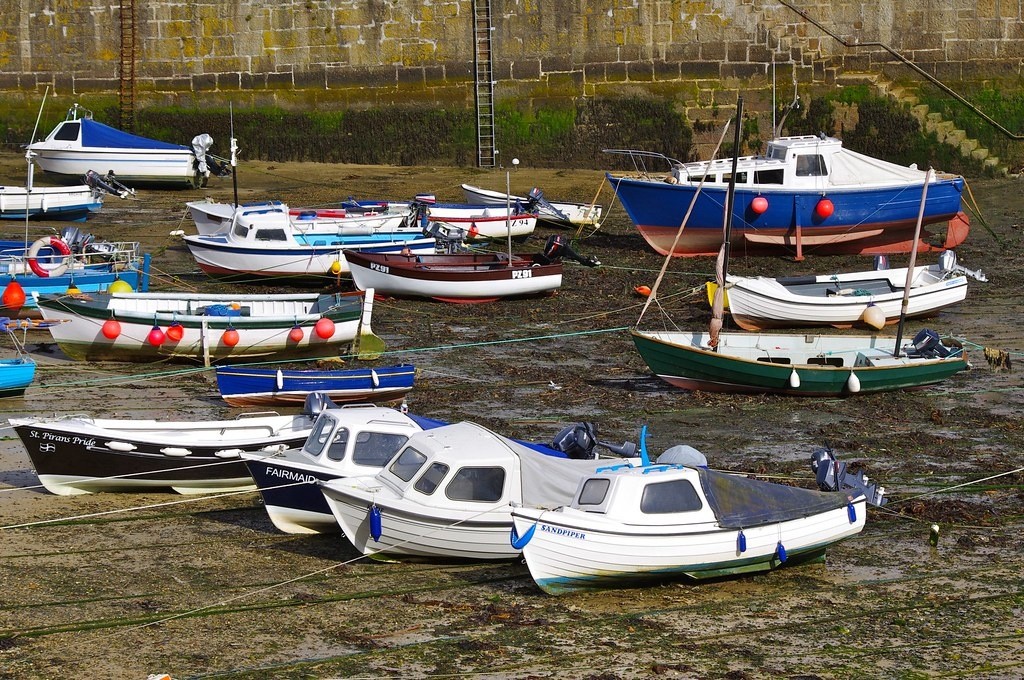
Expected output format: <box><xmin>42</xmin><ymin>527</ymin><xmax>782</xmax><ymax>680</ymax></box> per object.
<box><xmin>28</xmin><ymin>236</ymin><xmax>71</xmax><ymax>277</ymax></box>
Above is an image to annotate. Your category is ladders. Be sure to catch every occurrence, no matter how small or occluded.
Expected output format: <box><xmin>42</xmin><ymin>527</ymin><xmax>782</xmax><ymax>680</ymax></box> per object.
<box><xmin>119</xmin><ymin>0</ymin><xmax>136</xmax><ymax>133</ymax></box>
<box><xmin>475</xmin><ymin>0</ymin><xmax>497</xmax><ymax>167</ymax></box>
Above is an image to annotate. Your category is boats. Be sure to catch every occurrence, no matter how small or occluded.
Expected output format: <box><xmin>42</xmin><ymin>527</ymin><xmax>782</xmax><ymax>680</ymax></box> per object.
<box><xmin>720</xmin><ymin>249</ymin><xmax>989</xmax><ymax>331</ymax></box>
<box><xmin>0</xmin><ymin>170</ymin><xmax>141</xmax><ymax>223</ymax></box>
<box><xmin>166</xmin><ymin>100</ymin><xmax>570</xmax><ymax>282</ymax></box>
<box><xmin>0</xmin><ymin>315</ymin><xmax>73</xmax><ymax>395</ymax></box>
<box><xmin>341</xmin><ymin>171</ymin><xmax>602</xmax><ymax>305</ymax></box>
<box><xmin>628</xmin><ymin>95</ymin><xmax>974</xmax><ymax>398</ymax></box>
<box><xmin>0</xmin><ymin>86</ymin><xmax>151</xmax><ymax>310</ymax></box>
<box><xmin>702</xmin><ymin>252</ymin><xmax>890</xmax><ymax>317</ymax></box>
<box><xmin>602</xmin><ymin>58</ymin><xmax>971</xmax><ymax>259</ymax></box>
<box><xmin>30</xmin><ymin>283</ymin><xmax>387</xmax><ymax>362</ymax></box>
<box><xmin>505</xmin><ymin>426</ymin><xmax>890</xmax><ymax>596</ymax></box>
<box><xmin>313</xmin><ymin>418</ymin><xmax>654</xmax><ymax>565</ymax></box>
<box><xmin>213</xmin><ymin>364</ymin><xmax>416</xmax><ymax>410</ymax></box>
<box><xmin>6</xmin><ymin>388</ymin><xmax>342</xmax><ymax>498</ymax></box>
<box><xmin>19</xmin><ymin>101</ymin><xmax>232</xmax><ymax>190</ymax></box>
<box><xmin>238</xmin><ymin>404</ymin><xmax>457</xmax><ymax>536</ymax></box>
<box><xmin>460</xmin><ymin>182</ymin><xmax>604</xmax><ymax>226</ymax></box>
